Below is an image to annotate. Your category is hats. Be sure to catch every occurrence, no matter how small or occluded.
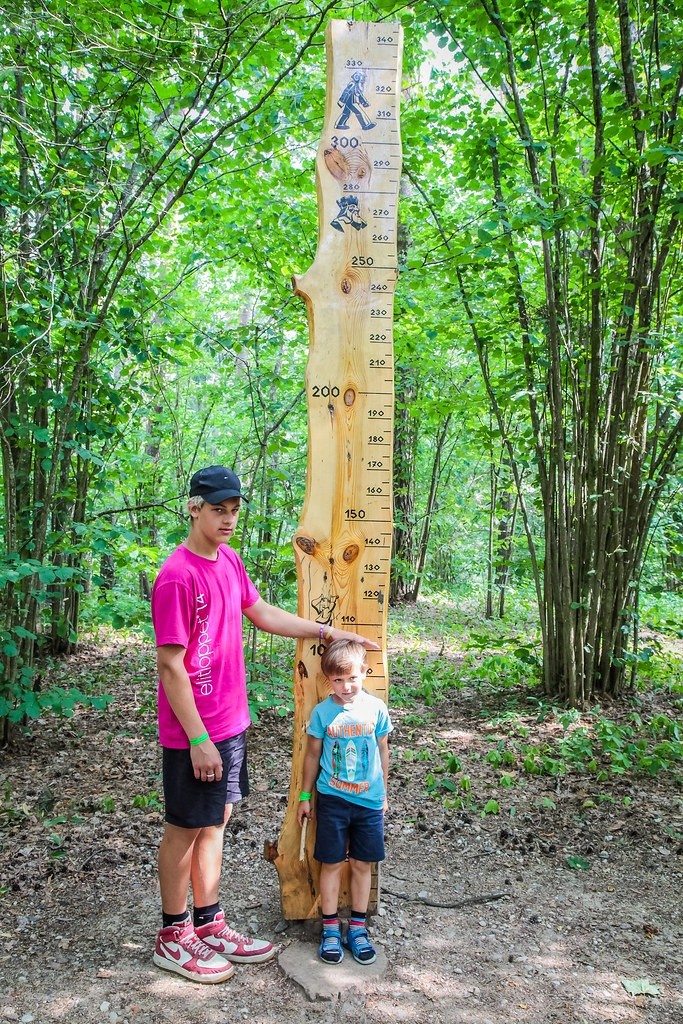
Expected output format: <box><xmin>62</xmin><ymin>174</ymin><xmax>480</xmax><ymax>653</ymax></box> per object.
<box><xmin>189</xmin><ymin>465</ymin><xmax>249</xmax><ymax>505</ymax></box>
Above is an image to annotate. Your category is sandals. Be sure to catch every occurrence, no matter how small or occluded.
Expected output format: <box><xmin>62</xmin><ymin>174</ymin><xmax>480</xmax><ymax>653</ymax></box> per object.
<box><xmin>319</xmin><ymin>928</ymin><xmax>344</xmax><ymax>964</ymax></box>
<box><xmin>342</xmin><ymin>928</ymin><xmax>376</xmax><ymax>964</ymax></box>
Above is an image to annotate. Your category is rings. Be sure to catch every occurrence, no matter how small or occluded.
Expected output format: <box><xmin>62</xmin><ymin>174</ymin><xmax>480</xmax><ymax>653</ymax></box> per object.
<box><xmin>207</xmin><ymin>774</ymin><xmax>214</xmax><ymax>778</ymax></box>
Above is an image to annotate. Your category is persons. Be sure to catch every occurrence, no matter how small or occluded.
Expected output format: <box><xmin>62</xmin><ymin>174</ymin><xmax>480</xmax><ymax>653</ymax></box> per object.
<box><xmin>153</xmin><ymin>467</ymin><xmax>380</xmax><ymax>983</ymax></box>
<box><xmin>296</xmin><ymin>638</ymin><xmax>394</xmax><ymax>966</ymax></box>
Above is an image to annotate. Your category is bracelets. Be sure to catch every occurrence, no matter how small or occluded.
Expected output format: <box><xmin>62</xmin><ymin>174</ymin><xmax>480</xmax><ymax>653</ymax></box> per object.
<box><xmin>300</xmin><ymin>791</ymin><xmax>311</xmax><ymax>803</ymax></box>
<box><xmin>319</xmin><ymin>624</ymin><xmax>334</xmax><ymax>640</ymax></box>
<box><xmin>189</xmin><ymin>732</ymin><xmax>210</xmax><ymax>746</ymax></box>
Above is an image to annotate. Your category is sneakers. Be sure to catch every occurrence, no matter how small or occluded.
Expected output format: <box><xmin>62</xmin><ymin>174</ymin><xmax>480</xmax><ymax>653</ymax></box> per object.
<box><xmin>194</xmin><ymin>908</ymin><xmax>274</xmax><ymax>963</ymax></box>
<box><xmin>153</xmin><ymin>910</ymin><xmax>234</xmax><ymax>983</ymax></box>
